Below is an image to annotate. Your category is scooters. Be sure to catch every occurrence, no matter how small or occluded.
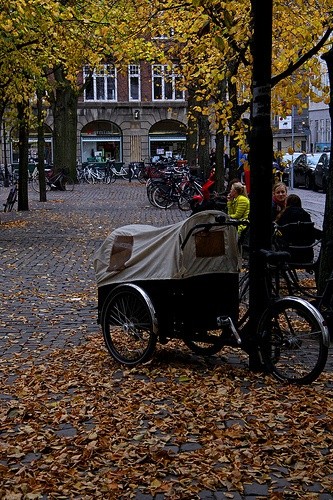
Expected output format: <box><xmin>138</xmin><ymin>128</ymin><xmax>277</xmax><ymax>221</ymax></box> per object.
<box><xmin>188</xmin><ymin>174</ymin><xmax>243</xmax><ymax>210</ymax></box>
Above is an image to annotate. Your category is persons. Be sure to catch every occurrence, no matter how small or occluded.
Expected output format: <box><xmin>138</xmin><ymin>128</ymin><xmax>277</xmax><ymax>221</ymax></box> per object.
<box><xmin>225</xmin><ymin>182</ymin><xmax>250</xmax><ymax>239</ymax></box>
<box><xmin>273</xmin><ymin>183</ymin><xmax>290</xmax><ymax>221</ymax></box>
<box><xmin>273</xmin><ymin>194</ymin><xmax>315</xmax><ymax>266</ymax></box>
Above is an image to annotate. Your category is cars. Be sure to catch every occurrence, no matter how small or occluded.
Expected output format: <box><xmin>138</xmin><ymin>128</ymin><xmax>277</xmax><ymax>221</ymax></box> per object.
<box><xmin>274</xmin><ymin>151</ymin><xmax>303</xmax><ymax>186</ymax></box>
<box><xmin>309</xmin><ymin>152</ymin><xmax>333</xmax><ymax>192</ymax></box>
<box><xmin>284</xmin><ymin>151</ymin><xmax>326</xmax><ymax>190</ymax></box>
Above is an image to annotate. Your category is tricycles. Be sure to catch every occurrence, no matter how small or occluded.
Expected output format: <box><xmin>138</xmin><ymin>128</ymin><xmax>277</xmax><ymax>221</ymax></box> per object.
<box><xmin>88</xmin><ymin>210</ymin><xmax>329</xmax><ymax>377</ymax></box>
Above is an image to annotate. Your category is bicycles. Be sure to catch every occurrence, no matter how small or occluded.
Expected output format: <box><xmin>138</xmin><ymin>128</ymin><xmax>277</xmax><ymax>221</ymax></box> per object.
<box><xmin>30</xmin><ymin>155</ymin><xmax>210</xmax><ymax>211</ymax></box>
<box><xmin>2</xmin><ymin>170</ymin><xmax>19</xmax><ymax>214</ymax></box>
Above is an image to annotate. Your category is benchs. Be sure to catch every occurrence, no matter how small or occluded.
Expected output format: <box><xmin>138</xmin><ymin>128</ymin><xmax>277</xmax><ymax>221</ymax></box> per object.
<box><xmin>274</xmin><ymin>228</ymin><xmax>324</xmax><ymax>294</ymax></box>
